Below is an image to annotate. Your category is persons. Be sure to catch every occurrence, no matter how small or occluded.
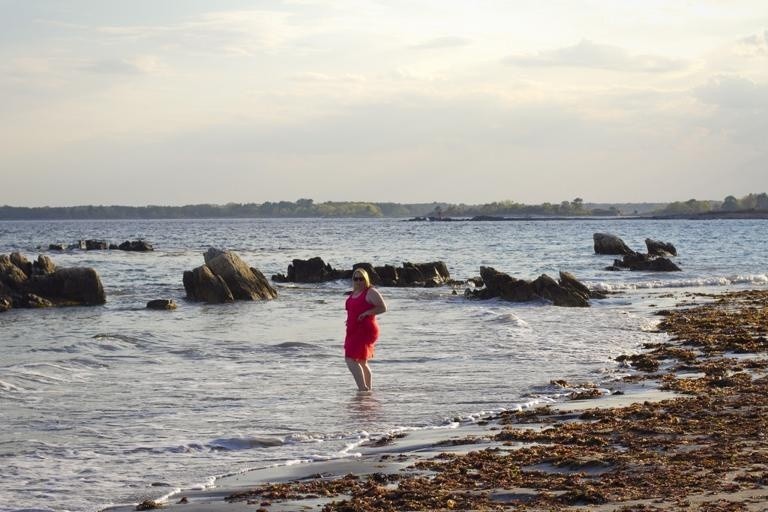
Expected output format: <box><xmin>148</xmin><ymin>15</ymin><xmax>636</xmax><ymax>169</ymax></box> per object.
<box><xmin>344</xmin><ymin>268</ymin><xmax>386</xmax><ymax>391</ymax></box>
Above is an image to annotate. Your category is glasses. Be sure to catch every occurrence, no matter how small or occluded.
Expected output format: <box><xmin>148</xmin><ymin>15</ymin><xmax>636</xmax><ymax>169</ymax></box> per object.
<box><xmin>353</xmin><ymin>277</ymin><xmax>365</xmax><ymax>281</ymax></box>
